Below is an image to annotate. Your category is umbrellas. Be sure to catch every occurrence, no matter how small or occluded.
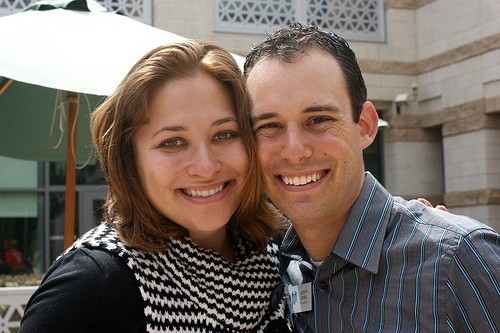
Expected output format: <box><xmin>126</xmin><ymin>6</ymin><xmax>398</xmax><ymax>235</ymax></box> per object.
<box><xmin>0</xmin><ymin>0</ymin><xmax>245</xmax><ymax>256</ymax></box>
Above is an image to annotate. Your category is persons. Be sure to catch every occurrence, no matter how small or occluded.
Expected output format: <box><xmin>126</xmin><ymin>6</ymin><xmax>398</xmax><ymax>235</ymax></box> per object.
<box><xmin>243</xmin><ymin>23</ymin><xmax>500</xmax><ymax>333</ymax></box>
<box><xmin>19</xmin><ymin>42</ymin><xmax>448</xmax><ymax>333</ymax></box>
